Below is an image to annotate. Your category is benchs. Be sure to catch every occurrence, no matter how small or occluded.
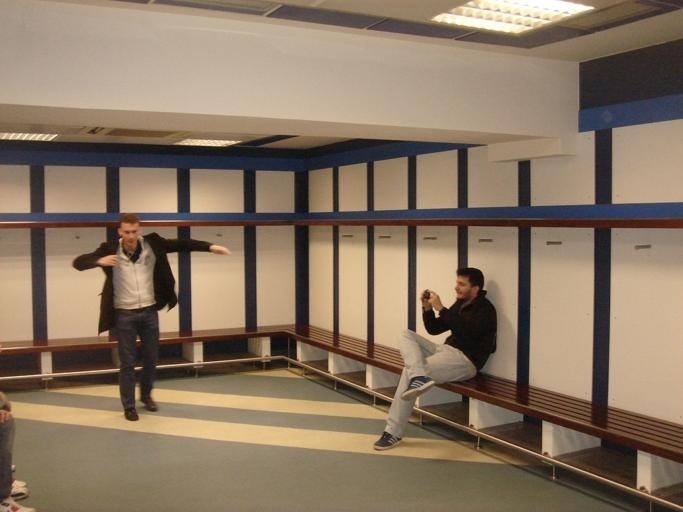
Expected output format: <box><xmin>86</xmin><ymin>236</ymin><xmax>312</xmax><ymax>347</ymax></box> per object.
<box><xmin>287</xmin><ymin>325</ymin><xmax>683</xmax><ymax>512</ymax></box>
<box><xmin>0</xmin><ymin>323</ymin><xmax>296</xmax><ymax>388</ymax></box>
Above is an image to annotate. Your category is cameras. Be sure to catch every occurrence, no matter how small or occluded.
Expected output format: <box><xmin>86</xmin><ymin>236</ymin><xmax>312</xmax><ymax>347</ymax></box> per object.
<box><xmin>423</xmin><ymin>290</ymin><xmax>430</xmax><ymax>299</ymax></box>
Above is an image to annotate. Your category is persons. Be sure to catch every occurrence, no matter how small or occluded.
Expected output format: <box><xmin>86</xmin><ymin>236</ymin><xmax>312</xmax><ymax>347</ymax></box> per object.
<box><xmin>0</xmin><ymin>389</ymin><xmax>40</xmax><ymax>512</ymax></box>
<box><xmin>71</xmin><ymin>211</ymin><xmax>232</xmax><ymax>423</ymax></box>
<box><xmin>370</xmin><ymin>267</ymin><xmax>499</xmax><ymax>451</ymax></box>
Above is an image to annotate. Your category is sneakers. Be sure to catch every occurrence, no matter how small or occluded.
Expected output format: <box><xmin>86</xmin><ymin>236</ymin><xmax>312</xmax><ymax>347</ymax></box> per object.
<box><xmin>400</xmin><ymin>374</ymin><xmax>436</xmax><ymax>401</ymax></box>
<box><xmin>123</xmin><ymin>407</ymin><xmax>138</xmax><ymax>422</ymax></box>
<box><xmin>143</xmin><ymin>397</ymin><xmax>161</xmax><ymax>412</ymax></box>
<box><xmin>373</xmin><ymin>430</ymin><xmax>403</xmax><ymax>451</ymax></box>
<box><xmin>0</xmin><ymin>463</ymin><xmax>37</xmax><ymax>512</ymax></box>
